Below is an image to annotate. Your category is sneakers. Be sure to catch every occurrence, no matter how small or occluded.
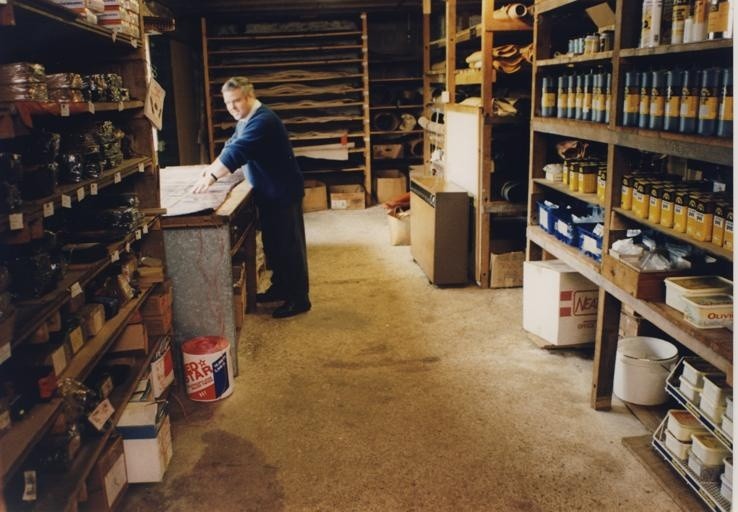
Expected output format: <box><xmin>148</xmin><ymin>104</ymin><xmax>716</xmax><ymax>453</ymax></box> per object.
<box><xmin>256</xmin><ymin>284</ymin><xmax>286</xmax><ymax>302</ymax></box>
<box><xmin>271</xmin><ymin>295</ymin><xmax>313</xmax><ymax>318</ymax></box>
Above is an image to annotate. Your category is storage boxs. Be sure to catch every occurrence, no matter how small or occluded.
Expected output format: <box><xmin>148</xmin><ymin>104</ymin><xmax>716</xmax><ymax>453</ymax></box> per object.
<box><xmin>299</xmin><ymin>177</ymin><xmax>366</xmax><ymax>214</ymax></box>
<box><xmin>81</xmin><ymin>399</ymin><xmax>173</xmax><ymax>509</ymax></box>
<box><xmin>518</xmin><ymin>259</ymin><xmax>601</xmax><ymax>347</ymax></box>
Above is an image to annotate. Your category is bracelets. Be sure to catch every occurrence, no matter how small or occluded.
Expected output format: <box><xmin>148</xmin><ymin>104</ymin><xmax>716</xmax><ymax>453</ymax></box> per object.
<box><xmin>210</xmin><ymin>171</ymin><xmax>218</xmax><ymax>182</ymax></box>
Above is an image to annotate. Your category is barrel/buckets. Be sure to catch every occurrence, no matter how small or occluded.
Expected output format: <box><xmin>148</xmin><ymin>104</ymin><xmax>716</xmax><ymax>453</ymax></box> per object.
<box><xmin>182</xmin><ymin>336</ymin><xmax>234</xmax><ymax>401</ymax></box>
<box><xmin>613</xmin><ymin>336</ymin><xmax>679</xmax><ymax>407</ymax></box>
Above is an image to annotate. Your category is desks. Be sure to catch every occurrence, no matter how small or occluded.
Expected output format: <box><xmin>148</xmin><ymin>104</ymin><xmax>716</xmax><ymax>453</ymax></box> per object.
<box><xmin>160</xmin><ymin>164</ymin><xmax>259</xmax><ymax>383</ymax></box>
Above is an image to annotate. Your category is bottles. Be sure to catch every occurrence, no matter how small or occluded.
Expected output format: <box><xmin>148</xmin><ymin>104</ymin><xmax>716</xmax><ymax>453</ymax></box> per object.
<box><xmin>540</xmin><ymin>1</ymin><xmax>733</xmax><ymax>141</ymax></box>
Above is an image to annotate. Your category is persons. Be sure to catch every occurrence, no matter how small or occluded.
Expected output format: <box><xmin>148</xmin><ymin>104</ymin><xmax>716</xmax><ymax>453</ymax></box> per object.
<box><xmin>192</xmin><ymin>77</ymin><xmax>312</xmax><ymax>318</ymax></box>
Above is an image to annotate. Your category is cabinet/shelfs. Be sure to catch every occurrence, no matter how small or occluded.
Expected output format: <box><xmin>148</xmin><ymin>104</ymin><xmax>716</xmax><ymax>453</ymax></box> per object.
<box><xmin>525</xmin><ymin>0</ymin><xmax>733</xmax><ymax>472</ymax></box>
<box><xmin>0</xmin><ymin>25</ymin><xmax>167</xmax><ymax>512</ymax></box>
<box><xmin>197</xmin><ymin>17</ymin><xmax>370</xmax><ymax>202</ymax></box>
<box><xmin>370</xmin><ymin>0</ymin><xmax>424</xmax><ymax>199</ymax></box>
<box><xmin>423</xmin><ymin>0</ymin><xmax>534</xmax><ymax>288</ymax></box>
